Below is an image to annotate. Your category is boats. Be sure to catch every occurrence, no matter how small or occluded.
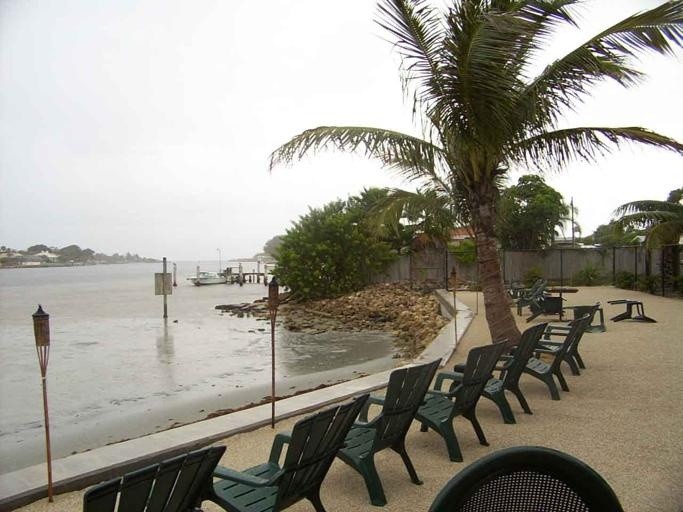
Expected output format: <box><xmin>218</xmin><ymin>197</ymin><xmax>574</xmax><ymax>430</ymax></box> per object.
<box><xmin>189</xmin><ymin>271</ymin><xmax>227</xmax><ymax>285</ymax></box>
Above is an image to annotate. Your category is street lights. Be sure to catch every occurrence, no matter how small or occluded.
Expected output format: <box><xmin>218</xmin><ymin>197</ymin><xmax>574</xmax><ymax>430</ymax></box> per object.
<box><xmin>266</xmin><ymin>276</ymin><xmax>278</xmax><ymax>428</ymax></box>
<box><xmin>449</xmin><ymin>267</ymin><xmax>457</xmax><ymax>348</ymax></box>
<box><xmin>31</xmin><ymin>303</ymin><xmax>52</xmax><ymax>505</ymax></box>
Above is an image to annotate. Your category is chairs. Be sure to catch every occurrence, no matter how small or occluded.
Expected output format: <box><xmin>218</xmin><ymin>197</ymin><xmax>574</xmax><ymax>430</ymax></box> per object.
<box><xmin>542</xmin><ymin>314</ymin><xmax>591</xmax><ymax>374</ymax></box>
<box><xmin>196</xmin><ymin>395</ymin><xmax>372</xmax><ymax>512</ymax></box>
<box><xmin>449</xmin><ymin>321</ymin><xmax>548</xmax><ymax>426</ymax></box>
<box><xmin>81</xmin><ymin>445</ymin><xmax>228</xmax><ymax>512</ymax></box>
<box><xmin>499</xmin><ymin>316</ymin><xmax>586</xmax><ymax>402</ymax></box>
<box><xmin>427</xmin><ymin>444</ymin><xmax>624</xmax><ymax>512</ymax></box>
<box><xmin>413</xmin><ymin>339</ymin><xmax>509</xmax><ymax>463</ymax></box>
<box><xmin>305</xmin><ymin>358</ymin><xmax>444</xmax><ymax>507</ymax></box>
